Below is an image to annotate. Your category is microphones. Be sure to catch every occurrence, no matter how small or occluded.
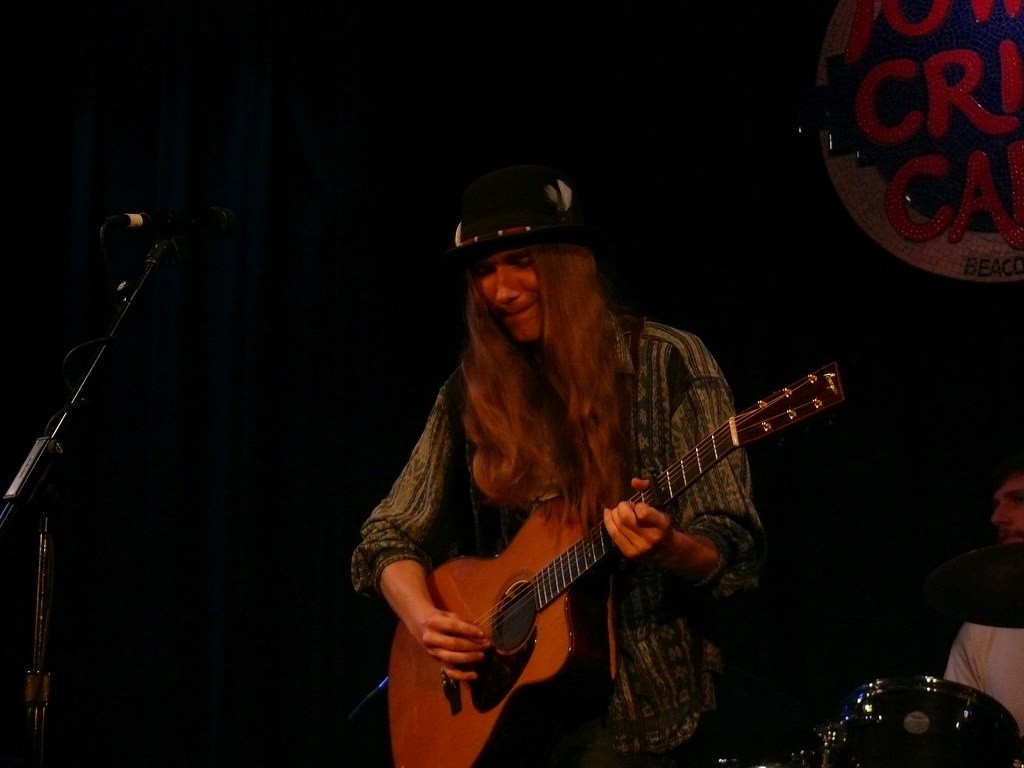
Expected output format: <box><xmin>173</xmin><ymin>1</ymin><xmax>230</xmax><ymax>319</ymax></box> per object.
<box><xmin>107</xmin><ymin>206</ymin><xmax>239</xmax><ymax>237</ymax></box>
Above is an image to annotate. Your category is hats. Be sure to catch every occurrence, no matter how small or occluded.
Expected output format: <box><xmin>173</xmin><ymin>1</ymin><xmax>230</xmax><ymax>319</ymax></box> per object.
<box><xmin>444</xmin><ymin>164</ymin><xmax>593</xmax><ymax>270</ymax></box>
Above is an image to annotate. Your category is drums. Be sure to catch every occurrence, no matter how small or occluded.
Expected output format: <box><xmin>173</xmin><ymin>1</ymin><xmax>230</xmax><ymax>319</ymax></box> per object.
<box><xmin>862</xmin><ymin>674</ymin><xmax>1020</xmax><ymax>766</ymax></box>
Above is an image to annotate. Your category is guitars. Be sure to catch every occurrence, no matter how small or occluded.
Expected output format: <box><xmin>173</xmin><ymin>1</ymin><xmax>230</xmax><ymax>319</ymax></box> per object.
<box><xmin>387</xmin><ymin>364</ymin><xmax>857</xmax><ymax>767</ymax></box>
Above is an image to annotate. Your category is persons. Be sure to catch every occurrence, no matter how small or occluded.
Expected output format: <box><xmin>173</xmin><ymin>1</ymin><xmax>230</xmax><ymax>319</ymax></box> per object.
<box><xmin>938</xmin><ymin>471</ymin><xmax>1024</xmax><ymax>761</ymax></box>
<box><xmin>351</xmin><ymin>154</ymin><xmax>761</xmax><ymax>768</ymax></box>
